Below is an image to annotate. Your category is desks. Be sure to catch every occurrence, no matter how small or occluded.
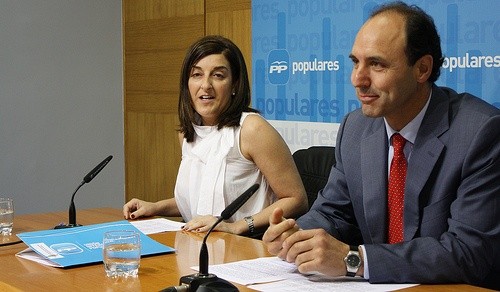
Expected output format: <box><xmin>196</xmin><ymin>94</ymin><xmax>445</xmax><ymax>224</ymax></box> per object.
<box><xmin>1</xmin><ymin>206</ymin><xmax>500</xmax><ymax>292</ymax></box>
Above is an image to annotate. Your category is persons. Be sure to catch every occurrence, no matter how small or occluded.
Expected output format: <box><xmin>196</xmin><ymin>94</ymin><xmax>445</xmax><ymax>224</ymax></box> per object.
<box><xmin>122</xmin><ymin>34</ymin><xmax>309</xmax><ymax>240</ymax></box>
<box><xmin>262</xmin><ymin>3</ymin><xmax>500</xmax><ymax>284</ymax></box>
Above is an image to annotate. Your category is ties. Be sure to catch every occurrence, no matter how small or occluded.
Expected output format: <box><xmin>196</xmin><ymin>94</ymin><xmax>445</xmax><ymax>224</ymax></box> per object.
<box><xmin>386</xmin><ymin>132</ymin><xmax>409</xmax><ymax>245</ymax></box>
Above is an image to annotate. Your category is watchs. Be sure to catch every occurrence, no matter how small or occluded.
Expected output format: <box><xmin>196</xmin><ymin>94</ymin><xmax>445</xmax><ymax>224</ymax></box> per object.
<box><xmin>245</xmin><ymin>216</ymin><xmax>256</xmax><ymax>234</ymax></box>
<box><xmin>342</xmin><ymin>243</ymin><xmax>362</xmax><ymax>277</ymax></box>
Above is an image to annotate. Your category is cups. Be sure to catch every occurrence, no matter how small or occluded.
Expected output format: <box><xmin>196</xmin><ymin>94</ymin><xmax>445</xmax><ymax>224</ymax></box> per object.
<box><xmin>0</xmin><ymin>197</ymin><xmax>14</xmax><ymax>233</ymax></box>
<box><xmin>103</xmin><ymin>230</ymin><xmax>141</xmax><ymax>278</ymax></box>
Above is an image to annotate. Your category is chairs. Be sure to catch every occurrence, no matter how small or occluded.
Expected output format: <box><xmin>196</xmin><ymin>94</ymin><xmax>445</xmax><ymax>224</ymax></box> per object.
<box><xmin>292</xmin><ymin>146</ymin><xmax>337</xmax><ymax>211</ymax></box>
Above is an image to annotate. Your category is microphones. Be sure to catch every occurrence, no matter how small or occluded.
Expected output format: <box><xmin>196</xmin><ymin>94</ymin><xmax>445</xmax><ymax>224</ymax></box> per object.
<box><xmin>54</xmin><ymin>155</ymin><xmax>113</xmax><ymax>229</ymax></box>
<box><xmin>179</xmin><ymin>184</ymin><xmax>260</xmax><ymax>292</ymax></box>
